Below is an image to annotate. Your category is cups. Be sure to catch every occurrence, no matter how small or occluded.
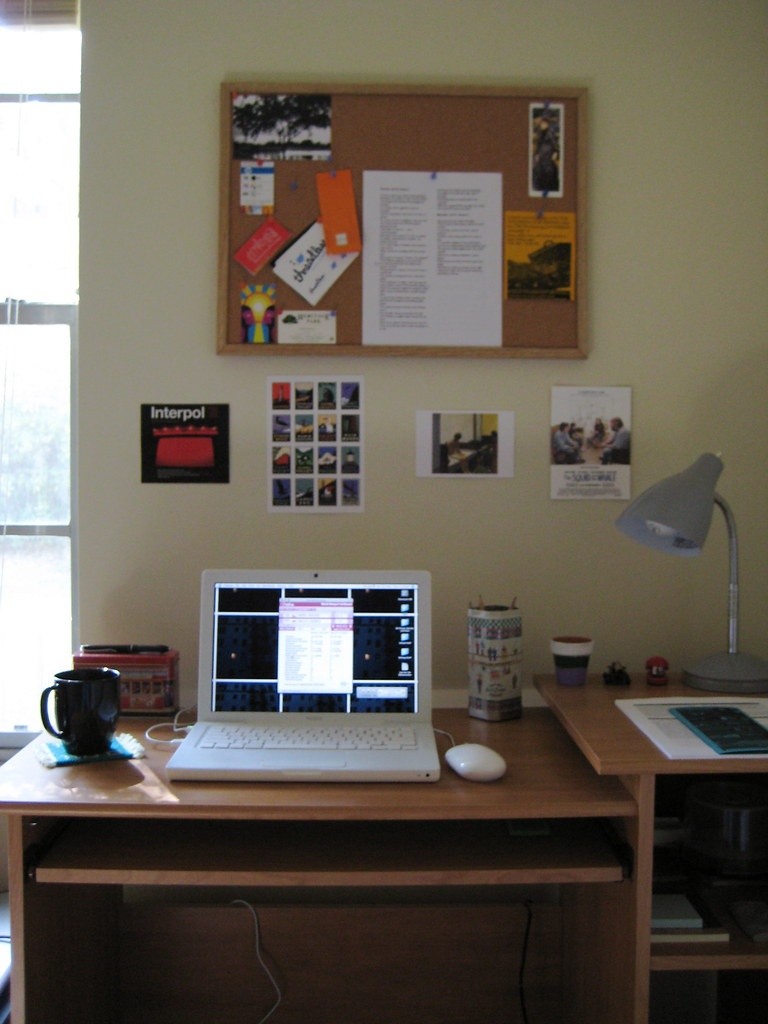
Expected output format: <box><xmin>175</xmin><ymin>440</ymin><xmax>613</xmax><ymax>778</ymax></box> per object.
<box><xmin>467</xmin><ymin>605</ymin><xmax>525</xmax><ymax>720</ymax></box>
<box><xmin>41</xmin><ymin>668</ymin><xmax>121</xmax><ymax>755</ymax></box>
<box><xmin>550</xmin><ymin>635</ymin><xmax>594</xmax><ymax>685</ymax></box>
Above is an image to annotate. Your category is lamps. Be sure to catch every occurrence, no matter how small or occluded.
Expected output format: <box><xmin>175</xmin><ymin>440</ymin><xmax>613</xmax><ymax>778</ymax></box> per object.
<box><xmin>619</xmin><ymin>450</ymin><xmax>768</xmax><ymax>681</ymax></box>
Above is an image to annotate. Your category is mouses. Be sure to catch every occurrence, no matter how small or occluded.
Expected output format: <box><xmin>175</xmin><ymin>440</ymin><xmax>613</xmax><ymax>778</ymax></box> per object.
<box><xmin>445</xmin><ymin>742</ymin><xmax>507</xmax><ymax>782</ymax></box>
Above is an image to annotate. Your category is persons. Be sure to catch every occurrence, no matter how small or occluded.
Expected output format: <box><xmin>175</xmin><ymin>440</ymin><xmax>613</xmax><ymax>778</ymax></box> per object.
<box><xmin>553</xmin><ymin>417</ymin><xmax>630</xmax><ymax>464</ymax></box>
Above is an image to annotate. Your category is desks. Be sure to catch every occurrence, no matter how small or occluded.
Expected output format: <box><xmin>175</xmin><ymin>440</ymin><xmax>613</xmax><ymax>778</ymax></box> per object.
<box><xmin>532</xmin><ymin>674</ymin><xmax>768</xmax><ymax>1024</ymax></box>
<box><xmin>0</xmin><ymin>708</ymin><xmax>636</xmax><ymax>1024</ymax></box>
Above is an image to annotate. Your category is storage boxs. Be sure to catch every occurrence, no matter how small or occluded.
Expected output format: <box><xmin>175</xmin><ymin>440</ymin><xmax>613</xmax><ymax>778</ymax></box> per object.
<box><xmin>72</xmin><ymin>645</ymin><xmax>180</xmax><ymax>719</ymax></box>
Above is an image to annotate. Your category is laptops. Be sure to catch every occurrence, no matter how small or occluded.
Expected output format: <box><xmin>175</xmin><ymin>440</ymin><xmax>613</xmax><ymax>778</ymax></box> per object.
<box><xmin>164</xmin><ymin>567</ymin><xmax>441</xmax><ymax>782</ymax></box>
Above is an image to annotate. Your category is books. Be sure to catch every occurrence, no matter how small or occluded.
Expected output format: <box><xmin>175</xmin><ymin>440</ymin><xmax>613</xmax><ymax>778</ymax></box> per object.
<box><xmin>650</xmin><ymin>886</ymin><xmax>768</xmax><ymax>943</ymax></box>
